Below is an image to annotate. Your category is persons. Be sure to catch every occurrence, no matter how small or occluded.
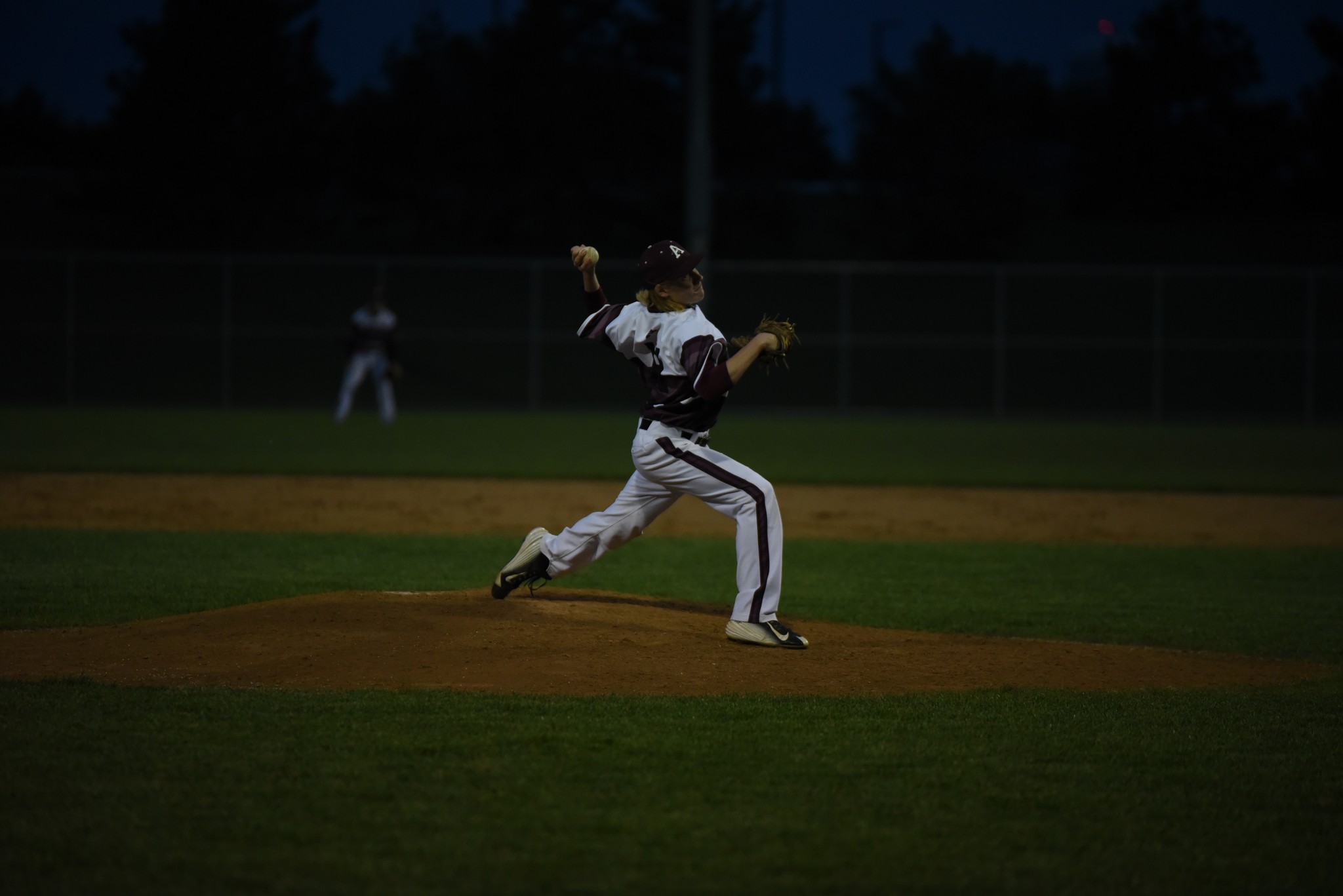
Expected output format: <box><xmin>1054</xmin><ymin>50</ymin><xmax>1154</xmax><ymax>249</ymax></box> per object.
<box><xmin>332</xmin><ymin>291</ymin><xmax>400</xmax><ymax>423</ymax></box>
<box><xmin>490</xmin><ymin>239</ymin><xmax>811</xmax><ymax>649</ymax></box>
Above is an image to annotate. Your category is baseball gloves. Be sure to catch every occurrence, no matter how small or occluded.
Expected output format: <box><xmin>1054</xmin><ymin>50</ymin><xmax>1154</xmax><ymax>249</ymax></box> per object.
<box><xmin>730</xmin><ymin>319</ymin><xmax>798</xmax><ymax>358</ymax></box>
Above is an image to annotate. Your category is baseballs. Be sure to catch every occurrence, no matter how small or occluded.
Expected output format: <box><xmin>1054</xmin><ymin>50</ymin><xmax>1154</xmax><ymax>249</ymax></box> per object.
<box><xmin>577</xmin><ymin>246</ymin><xmax>600</xmax><ymax>269</ymax></box>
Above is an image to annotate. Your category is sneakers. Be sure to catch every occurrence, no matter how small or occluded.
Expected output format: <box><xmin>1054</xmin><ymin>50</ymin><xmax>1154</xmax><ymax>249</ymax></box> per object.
<box><xmin>725</xmin><ymin>618</ymin><xmax>809</xmax><ymax>649</ymax></box>
<box><xmin>491</xmin><ymin>527</ymin><xmax>552</xmax><ymax>598</ymax></box>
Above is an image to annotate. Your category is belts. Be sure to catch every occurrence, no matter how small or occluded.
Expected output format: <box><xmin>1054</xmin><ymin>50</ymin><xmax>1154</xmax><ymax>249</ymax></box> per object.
<box><xmin>639</xmin><ymin>417</ymin><xmax>710</xmax><ymax>447</ymax></box>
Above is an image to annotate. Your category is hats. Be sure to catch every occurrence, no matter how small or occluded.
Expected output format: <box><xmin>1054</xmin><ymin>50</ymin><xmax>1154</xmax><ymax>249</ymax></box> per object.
<box><xmin>638</xmin><ymin>240</ymin><xmax>705</xmax><ymax>288</ymax></box>
